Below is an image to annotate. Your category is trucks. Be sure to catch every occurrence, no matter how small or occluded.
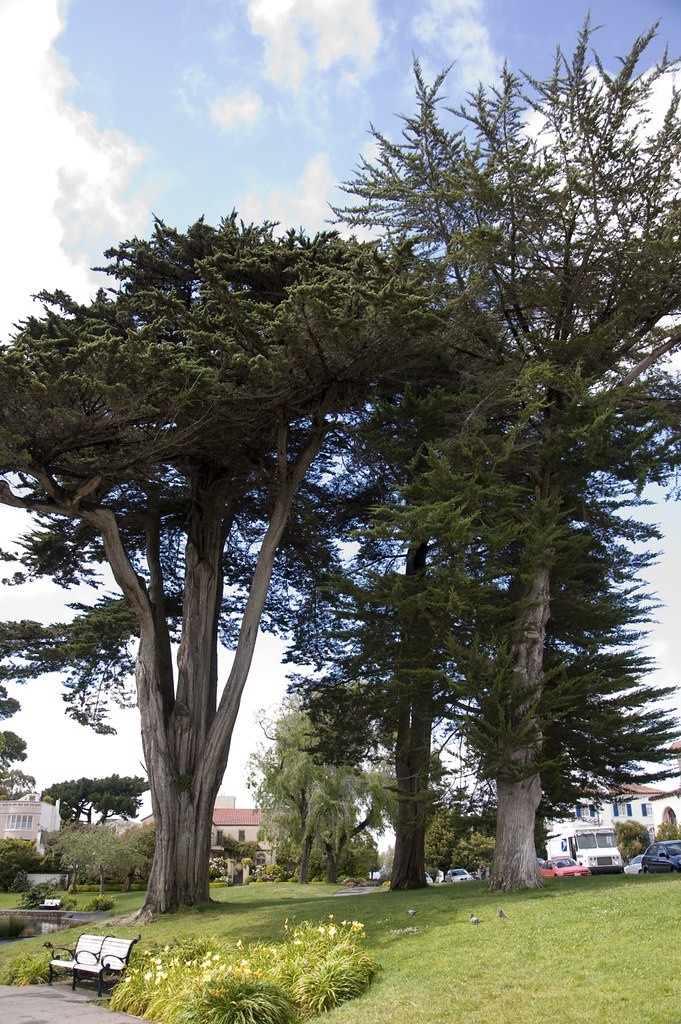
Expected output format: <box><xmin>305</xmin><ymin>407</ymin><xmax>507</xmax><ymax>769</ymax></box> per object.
<box><xmin>551</xmin><ymin>827</ymin><xmax>624</xmax><ymax>874</ymax></box>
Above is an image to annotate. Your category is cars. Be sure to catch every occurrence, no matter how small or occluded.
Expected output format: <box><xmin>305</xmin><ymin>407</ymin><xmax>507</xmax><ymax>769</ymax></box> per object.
<box><xmin>624</xmin><ymin>854</ymin><xmax>644</xmax><ymax>875</ymax></box>
<box><xmin>425</xmin><ymin>872</ymin><xmax>433</xmax><ymax>883</ymax></box>
<box><xmin>641</xmin><ymin>839</ymin><xmax>681</xmax><ymax>872</ymax></box>
<box><xmin>444</xmin><ymin>869</ymin><xmax>473</xmax><ymax>882</ymax></box>
<box><xmin>540</xmin><ymin>857</ymin><xmax>590</xmax><ymax>877</ymax></box>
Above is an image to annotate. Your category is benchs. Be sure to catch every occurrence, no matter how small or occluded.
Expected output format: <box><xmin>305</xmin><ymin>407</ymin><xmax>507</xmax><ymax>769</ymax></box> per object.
<box><xmin>39</xmin><ymin>899</ymin><xmax>62</xmax><ymax>911</ymax></box>
<box><xmin>48</xmin><ymin>932</ymin><xmax>142</xmax><ymax>998</ymax></box>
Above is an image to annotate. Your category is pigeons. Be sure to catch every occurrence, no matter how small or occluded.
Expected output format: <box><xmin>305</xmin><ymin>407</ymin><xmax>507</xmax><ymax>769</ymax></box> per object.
<box><xmin>406</xmin><ymin>909</ymin><xmax>506</xmax><ymax>925</ymax></box>
